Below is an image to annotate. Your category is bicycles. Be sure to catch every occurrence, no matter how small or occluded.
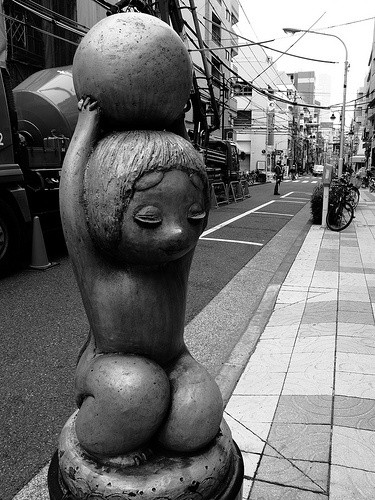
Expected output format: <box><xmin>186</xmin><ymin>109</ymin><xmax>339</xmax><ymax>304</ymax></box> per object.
<box><xmin>326</xmin><ymin>172</ymin><xmax>361</xmax><ymax>232</ymax></box>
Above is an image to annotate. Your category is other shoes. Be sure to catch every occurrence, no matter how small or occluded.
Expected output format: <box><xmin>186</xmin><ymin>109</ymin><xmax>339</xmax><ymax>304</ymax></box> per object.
<box><xmin>274</xmin><ymin>193</ymin><xmax>280</xmax><ymax>196</ymax></box>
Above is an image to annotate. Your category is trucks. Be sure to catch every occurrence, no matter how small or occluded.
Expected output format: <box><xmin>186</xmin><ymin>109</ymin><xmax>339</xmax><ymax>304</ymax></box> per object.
<box><xmin>186</xmin><ymin>128</ymin><xmax>241</xmax><ymax>196</ymax></box>
<box><xmin>1</xmin><ymin>65</ymin><xmax>79</xmax><ymax>274</ymax></box>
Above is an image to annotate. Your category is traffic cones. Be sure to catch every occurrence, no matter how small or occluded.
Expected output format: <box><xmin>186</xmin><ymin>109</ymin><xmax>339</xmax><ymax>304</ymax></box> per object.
<box><xmin>28</xmin><ymin>215</ymin><xmax>59</xmax><ymax>271</ymax></box>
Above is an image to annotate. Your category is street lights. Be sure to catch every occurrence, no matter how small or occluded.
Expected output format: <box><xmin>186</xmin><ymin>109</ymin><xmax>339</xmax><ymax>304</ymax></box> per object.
<box><xmin>282</xmin><ymin>27</ymin><xmax>348</xmax><ymax>178</ymax></box>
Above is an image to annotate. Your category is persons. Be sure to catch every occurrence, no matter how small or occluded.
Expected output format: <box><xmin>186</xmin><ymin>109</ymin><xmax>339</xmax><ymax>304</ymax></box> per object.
<box><xmin>290</xmin><ymin>165</ymin><xmax>298</xmax><ymax>180</ymax></box>
<box><xmin>348</xmin><ymin>166</ymin><xmax>354</xmax><ymax>175</ymax></box>
<box><xmin>57</xmin><ymin>92</ymin><xmax>224</xmax><ymax>456</ymax></box>
<box><xmin>273</xmin><ymin>160</ymin><xmax>282</xmax><ymax>195</ymax></box>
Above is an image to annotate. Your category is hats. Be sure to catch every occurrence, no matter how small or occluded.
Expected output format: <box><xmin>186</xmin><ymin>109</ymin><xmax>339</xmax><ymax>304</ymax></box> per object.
<box><xmin>277</xmin><ymin>159</ymin><xmax>282</xmax><ymax>164</ymax></box>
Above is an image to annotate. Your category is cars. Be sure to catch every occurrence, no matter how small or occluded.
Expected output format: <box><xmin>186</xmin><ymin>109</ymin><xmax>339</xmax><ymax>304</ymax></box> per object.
<box><xmin>312</xmin><ymin>164</ymin><xmax>325</xmax><ymax>177</ymax></box>
